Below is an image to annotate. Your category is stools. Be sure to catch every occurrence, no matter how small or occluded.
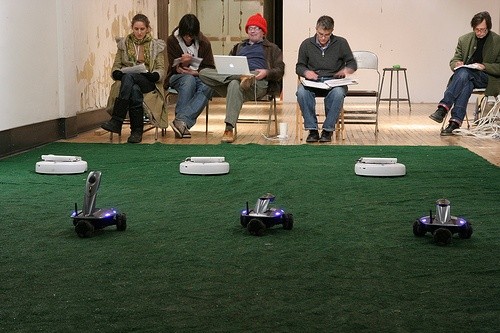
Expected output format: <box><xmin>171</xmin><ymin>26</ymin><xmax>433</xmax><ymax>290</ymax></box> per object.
<box><xmin>443</xmin><ymin>87</ymin><xmax>499</xmax><ymax>133</ymax></box>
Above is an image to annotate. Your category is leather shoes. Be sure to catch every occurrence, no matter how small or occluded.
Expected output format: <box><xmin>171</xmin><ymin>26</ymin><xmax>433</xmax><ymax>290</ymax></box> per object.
<box><xmin>440</xmin><ymin>121</ymin><xmax>459</xmax><ymax>135</ymax></box>
<box><xmin>429</xmin><ymin>109</ymin><xmax>445</xmax><ymax>123</ymax></box>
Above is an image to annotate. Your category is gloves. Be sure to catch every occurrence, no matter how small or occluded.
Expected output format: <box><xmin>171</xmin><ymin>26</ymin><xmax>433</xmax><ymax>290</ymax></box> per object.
<box><xmin>146</xmin><ymin>72</ymin><xmax>159</xmax><ymax>83</ymax></box>
<box><xmin>112</xmin><ymin>70</ymin><xmax>123</xmax><ymax>81</ymax></box>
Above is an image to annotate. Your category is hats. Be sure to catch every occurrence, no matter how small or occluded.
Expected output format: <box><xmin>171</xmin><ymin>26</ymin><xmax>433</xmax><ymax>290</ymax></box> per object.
<box><xmin>244</xmin><ymin>13</ymin><xmax>267</xmax><ymax>34</ymax></box>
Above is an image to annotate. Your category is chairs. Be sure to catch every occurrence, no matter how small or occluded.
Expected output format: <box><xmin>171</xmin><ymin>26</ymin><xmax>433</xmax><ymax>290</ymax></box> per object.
<box><xmin>295</xmin><ymin>50</ymin><xmax>380</xmax><ymax>141</ymax></box>
<box><xmin>110</xmin><ymin>61</ymin><xmax>285</xmax><ymax>141</ymax></box>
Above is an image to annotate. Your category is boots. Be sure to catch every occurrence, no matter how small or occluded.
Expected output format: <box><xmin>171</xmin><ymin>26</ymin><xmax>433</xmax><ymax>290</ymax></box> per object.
<box><xmin>101</xmin><ymin>97</ymin><xmax>129</xmax><ymax>134</ymax></box>
<box><xmin>128</xmin><ymin>106</ymin><xmax>144</xmax><ymax>143</ymax></box>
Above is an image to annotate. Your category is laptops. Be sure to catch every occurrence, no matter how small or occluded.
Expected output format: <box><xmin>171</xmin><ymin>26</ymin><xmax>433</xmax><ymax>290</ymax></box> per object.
<box><xmin>213</xmin><ymin>53</ymin><xmax>257</xmax><ymax>77</ymax></box>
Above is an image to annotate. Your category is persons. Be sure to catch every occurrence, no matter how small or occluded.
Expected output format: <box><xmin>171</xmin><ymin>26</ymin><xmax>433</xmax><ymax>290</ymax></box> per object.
<box><xmin>428</xmin><ymin>11</ymin><xmax>500</xmax><ymax>136</ymax></box>
<box><xmin>199</xmin><ymin>13</ymin><xmax>285</xmax><ymax>142</ymax></box>
<box><xmin>163</xmin><ymin>14</ymin><xmax>216</xmax><ymax>138</ymax></box>
<box><xmin>101</xmin><ymin>14</ymin><xmax>175</xmax><ymax>142</ymax></box>
<box><xmin>295</xmin><ymin>16</ymin><xmax>357</xmax><ymax>141</ymax></box>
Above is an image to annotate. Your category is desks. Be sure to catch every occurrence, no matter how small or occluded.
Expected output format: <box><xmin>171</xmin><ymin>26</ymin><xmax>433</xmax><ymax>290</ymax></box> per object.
<box><xmin>379</xmin><ymin>67</ymin><xmax>412</xmax><ymax>112</ymax></box>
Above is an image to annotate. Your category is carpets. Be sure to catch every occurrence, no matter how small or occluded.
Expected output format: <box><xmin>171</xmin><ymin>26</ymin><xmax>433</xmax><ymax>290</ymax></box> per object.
<box><xmin>0</xmin><ymin>140</ymin><xmax>499</xmax><ymax>332</ymax></box>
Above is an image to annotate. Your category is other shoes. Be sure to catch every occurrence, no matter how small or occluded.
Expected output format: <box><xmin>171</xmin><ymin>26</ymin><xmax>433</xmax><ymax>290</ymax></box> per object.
<box><xmin>306</xmin><ymin>132</ymin><xmax>320</xmax><ymax>142</ymax></box>
<box><xmin>320</xmin><ymin>130</ymin><xmax>333</xmax><ymax>142</ymax></box>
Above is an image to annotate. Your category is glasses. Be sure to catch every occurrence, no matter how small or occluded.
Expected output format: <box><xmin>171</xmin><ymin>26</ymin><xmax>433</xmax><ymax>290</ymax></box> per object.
<box><xmin>474</xmin><ymin>26</ymin><xmax>488</xmax><ymax>33</ymax></box>
<box><xmin>247</xmin><ymin>27</ymin><xmax>260</xmax><ymax>31</ymax></box>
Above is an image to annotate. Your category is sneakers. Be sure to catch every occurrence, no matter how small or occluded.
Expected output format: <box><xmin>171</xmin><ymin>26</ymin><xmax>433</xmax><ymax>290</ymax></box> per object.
<box><xmin>240</xmin><ymin>75</ymin><xmax>255</xmax><ymax>90</ymax></box>
<box><xmin>171</xmin><ymin>119</ymin><xmax>187</xmax><ymax>137</ymax></box>
<box><xmin>174</xmin><ymin>129</ymin><xmax>191</xmax><ymax>138</ymax></box>
<box><xmin>221</xmin><ymin>130</ymin><xmax>234</xmax><ymax>142</ymax></box>
